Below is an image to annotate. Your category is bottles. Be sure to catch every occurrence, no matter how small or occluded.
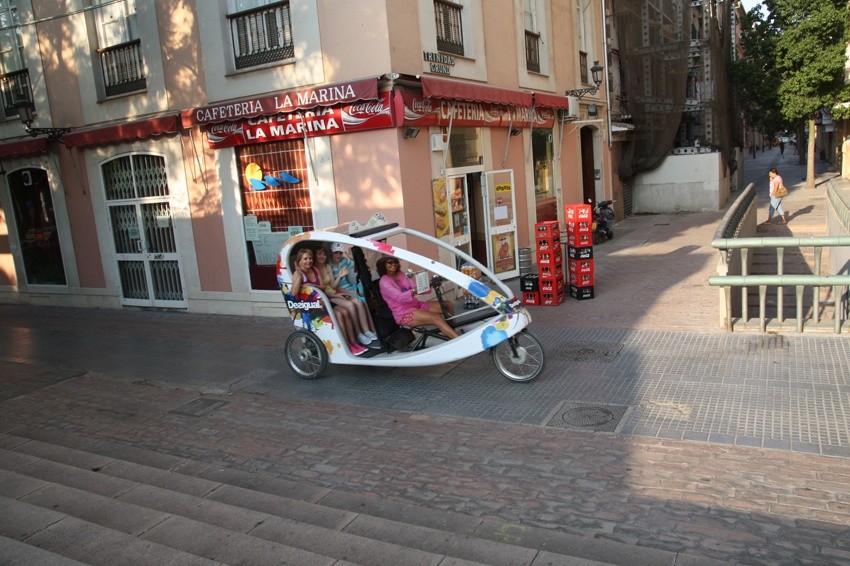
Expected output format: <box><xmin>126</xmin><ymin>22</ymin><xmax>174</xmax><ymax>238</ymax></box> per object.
<box><xmin>524</xmin><ymin>207</ymin><xmax>591</xmax><ymax>299</ymax></box>
<box><xmin>461</xmin><ymin>262</ymin><xmax>482</xmax><ymax>308</ymax></box>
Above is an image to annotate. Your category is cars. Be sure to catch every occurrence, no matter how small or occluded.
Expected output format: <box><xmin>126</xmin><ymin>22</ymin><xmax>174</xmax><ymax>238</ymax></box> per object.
<box><xmin>778</xmin><ymin>135</ymin><xmax>797</xmax><ymax>144</ymax></box>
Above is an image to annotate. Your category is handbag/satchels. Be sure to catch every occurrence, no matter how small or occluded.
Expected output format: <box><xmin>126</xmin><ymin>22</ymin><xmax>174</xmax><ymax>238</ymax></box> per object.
<box><xmin>774</xmin><ymin>184</ymin><xmax>788</xmax><ymax>197</ymax></box>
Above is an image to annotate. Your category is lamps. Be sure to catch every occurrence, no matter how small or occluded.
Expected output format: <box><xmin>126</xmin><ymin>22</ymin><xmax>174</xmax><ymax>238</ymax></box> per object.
<box><xmin>510</xmin><ymin>129</ymin><xmax>522</xmax><ymax>137</ymax></box>
<box><xmin>565</xmin><ymin>61</ymin><xmax>603</xmax><ymax>100</ymax></box>
<box><xmin>587</xmin><ymin>104</ymin><xmax>598</xmax><ymax>117</ymax></box>
<box><xmin>405</xmin><ymin>128</ymin><xmax>420</xmax><ymax>139</ymax></box>
<box><xmin>12</xmin><ymin>94</ymin><xmax>71</xmax><ymax>144</ymax></box>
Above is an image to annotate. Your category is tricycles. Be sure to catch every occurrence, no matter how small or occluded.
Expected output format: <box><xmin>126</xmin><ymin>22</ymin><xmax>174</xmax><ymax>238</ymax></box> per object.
<box><xmin>276</xmin><ymin>223</ymin><xmax>546</xmax><ymax>385</ymax></box>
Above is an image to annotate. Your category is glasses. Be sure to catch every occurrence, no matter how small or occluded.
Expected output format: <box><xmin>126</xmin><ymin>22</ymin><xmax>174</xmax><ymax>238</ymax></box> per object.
<box><xmin>386</xmin><ymin>260</ymin><xmax>398</xmax><ymax>264</ymax></box>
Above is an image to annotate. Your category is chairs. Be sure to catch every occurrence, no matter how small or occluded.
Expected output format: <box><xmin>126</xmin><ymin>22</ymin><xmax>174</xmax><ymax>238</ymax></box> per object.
<box><xmin>365</xmin><ymin>279</ymin><xmax>415</xmax><ymax>351</ymax></box>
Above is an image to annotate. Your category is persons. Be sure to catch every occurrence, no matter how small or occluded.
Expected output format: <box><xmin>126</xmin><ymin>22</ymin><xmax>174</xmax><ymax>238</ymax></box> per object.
<box><xmin>375</xmin><ymin>256</ymin><xmax>459</xmax><ymax>338</ymax></box>
<box><xmin>779</xmin><ymin>140</ymin><xmax>785</xmax><ymax>154</ymax></box>
<box><xmin>291</xmin><ymin>241</ymin><xmax>377</xmax><ymax>356</ymax></box>
<box><xmin>761</xmin><ymin>168</ymin><xmax>786</xmax><ymax>225</ymax></box>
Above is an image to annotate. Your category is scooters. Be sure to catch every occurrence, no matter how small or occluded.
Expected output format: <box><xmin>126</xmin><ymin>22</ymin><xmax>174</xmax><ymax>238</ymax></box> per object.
<box><xmin>587</xmin><ymin>198</ymin><xmax>616</xmax><ymax>244</ymax></box>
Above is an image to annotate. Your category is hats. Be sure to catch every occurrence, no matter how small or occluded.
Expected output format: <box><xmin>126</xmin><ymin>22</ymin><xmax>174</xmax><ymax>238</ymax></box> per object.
<box><xmin>330</xmin><ymin>242</ymin><xmax>344</xmax><ymax>252</ymax></box>
<box><xmin>376</xmin><ymin>253</ymin><xmax>393</xmax><ymax>264</ymax></box>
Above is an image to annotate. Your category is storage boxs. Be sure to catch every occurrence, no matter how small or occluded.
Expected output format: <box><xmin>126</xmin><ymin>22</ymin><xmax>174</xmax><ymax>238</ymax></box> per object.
<box><xmin>519</xmin><ymin>204</ymin><xmax>595</xmax><ymax>307</ymax></box>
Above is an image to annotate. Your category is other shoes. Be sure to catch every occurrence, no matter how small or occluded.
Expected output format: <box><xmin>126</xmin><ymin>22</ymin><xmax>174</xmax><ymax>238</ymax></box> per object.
<box><xmin>357</xmin><ymin>334</ymin><xmax>372</xmax><ymax>345</ymax></box>
<box><xmin>365</xmin><ymin>332</ymin><xmax>377</xmax><ymax>340</ymax></box>
<box><xmin>353</xmin><ymin>342</ymin><xmax>369</xmax><ymax>352</ymax></box>
<box><xmin>349</xmin><ymin>345</ymin><xmax>364</xmax><ymax>355</ymax></box>
